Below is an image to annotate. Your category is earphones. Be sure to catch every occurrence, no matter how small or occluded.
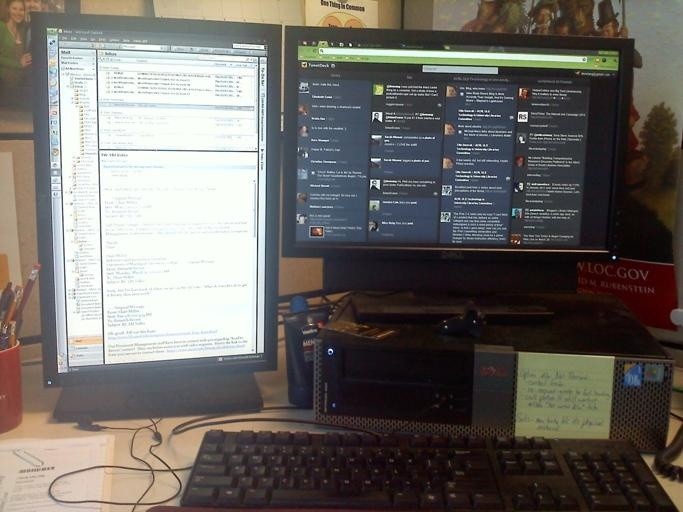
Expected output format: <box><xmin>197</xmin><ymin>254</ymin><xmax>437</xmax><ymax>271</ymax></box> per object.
<box><xmin>76</xmin><ymin>412</ymin><xmax>108</xmax><ymax>431</ymax></box>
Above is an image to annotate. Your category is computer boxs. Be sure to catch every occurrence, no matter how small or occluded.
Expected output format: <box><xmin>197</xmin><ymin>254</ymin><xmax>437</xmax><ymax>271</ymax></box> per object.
<box><xmin>311</xmin><ymin>288</ymin><xmax>676</xmax><ymax>455</ymax></box>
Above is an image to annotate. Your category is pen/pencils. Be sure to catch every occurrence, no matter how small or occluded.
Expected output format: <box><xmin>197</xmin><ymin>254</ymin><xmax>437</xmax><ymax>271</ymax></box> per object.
<box><xmin>0</xmin><ymin>261</ymin><xmax>41</xmax><ymax>351</ymax></box>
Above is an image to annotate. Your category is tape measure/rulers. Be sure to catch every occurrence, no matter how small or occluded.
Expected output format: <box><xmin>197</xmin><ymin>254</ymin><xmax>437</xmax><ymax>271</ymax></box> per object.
<box><xmin>0</xmin><ymin>254</ymin><xmax>11</xmax><ymax>294</ymax></box>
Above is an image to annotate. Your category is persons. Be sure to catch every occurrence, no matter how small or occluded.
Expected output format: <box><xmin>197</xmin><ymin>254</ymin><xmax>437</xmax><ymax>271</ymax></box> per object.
<box><xmin>21</xmin><ymin>1</ymin><xmax>44</xmax><ymax>70</ymax></box>
<box><xmin>0</xmin><ymin>1</ymin><xmax>31</xmax><ymax>70</ymax></box>
<box><xmin>526</xmin><ymin>0</ymin><xmax>643</xmax><ymax>70</ymax></box>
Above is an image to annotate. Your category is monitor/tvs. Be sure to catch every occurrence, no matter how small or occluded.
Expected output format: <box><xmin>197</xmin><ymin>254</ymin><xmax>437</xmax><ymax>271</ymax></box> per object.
<box><xmin>30</xmin><ymin>8</ymin><xmax>282</xmax><ymax>424</ymax></box>
<box><xmin>281</xmin><ymin>23</ymin><xmax>636</xmax><ymax>321</ymax></box>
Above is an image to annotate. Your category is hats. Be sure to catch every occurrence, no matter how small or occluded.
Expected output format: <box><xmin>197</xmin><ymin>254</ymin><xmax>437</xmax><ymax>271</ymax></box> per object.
<box><xmin>596</xmin><ymin>0</ymin><xmax>618</xmax><ymax>28</ymax></box>
<box><xmin>527</xmin><ymin>0</ymin><xmax>558</xmax><ymax>17</ymax></box>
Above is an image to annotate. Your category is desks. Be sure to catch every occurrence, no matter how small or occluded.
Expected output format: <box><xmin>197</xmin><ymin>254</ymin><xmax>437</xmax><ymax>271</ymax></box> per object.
<box><xmin>0</xmin><ymin>290</ymin><xmax>683</xmax><ymax>511</ymax></box>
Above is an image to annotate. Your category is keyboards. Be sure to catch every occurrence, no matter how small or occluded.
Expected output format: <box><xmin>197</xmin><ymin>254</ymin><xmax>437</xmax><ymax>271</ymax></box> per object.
<box><xmin>177</xmin><ymin>428</ymin><xmax>683</xmax><ymax>512</ymax></box>
